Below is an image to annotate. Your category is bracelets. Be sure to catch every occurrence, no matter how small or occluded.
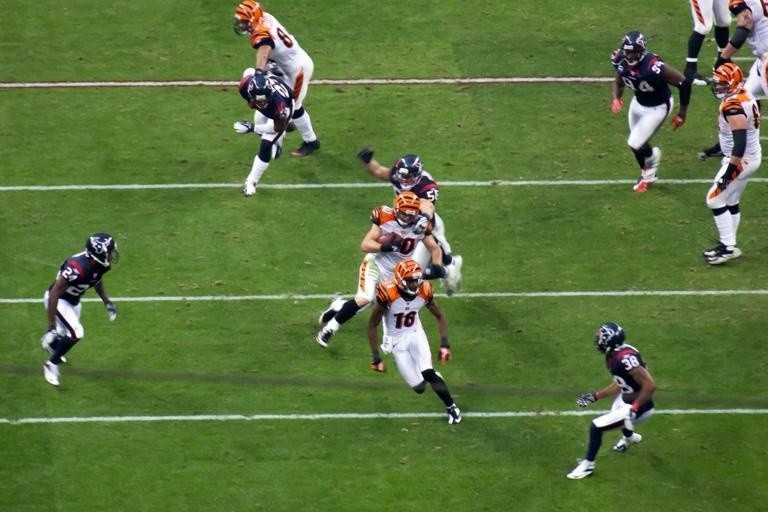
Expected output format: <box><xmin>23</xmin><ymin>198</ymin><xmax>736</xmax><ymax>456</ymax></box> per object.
<box><xmin>440</xmin><ymin>337</ymin><xmax>448</xmax><ymax>345</ymax></box>
<box><xmin>678</xmin><ymin>114</ymin><xmax>686</xmax><ymax>119</ymax></box>
<box><xmin>373</xmin><ymin>351</ymin><xmax>380</xmax><ymax>358</ymax></box>
<box><xmin>102</xmin><ymin>298</ymin><xmax>108</xmax><ymax>300</ymax></box>
<box><xmin>631</xmin><ymin>402</ymin><xmax>640</xmax><ymax>411</ymax></box>
<box><xmin>48</xmin><ymin>325</ymin><xmax>55</xmax><ymax>328</ymax></box>
<box><xmin>596</xmin><ymin>391</ymin><xmax>605</xmax><ymax>399</ymax></box>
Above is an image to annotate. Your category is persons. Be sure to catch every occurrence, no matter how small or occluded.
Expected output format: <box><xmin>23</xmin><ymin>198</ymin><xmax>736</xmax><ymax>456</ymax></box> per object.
<box><xmin>682</xmin><ymin>0</ymin><xmax>732</xmax><ymax>86</ymax></box>
<box><xmin>609</xmin><ymin>30</ymin><xmax>691</xmax><ymax>192</ymax></box>
<box><xmin>566</xmin><ymin>321</ymin><xmax>656</xmax><ymax>479</ymax></box>
<box><xmin>232</xmin><ymin>0</ymin><xmax>320</xmax><ymax>157</ymax></box>
<box><xmin>697</xmin><ymin>63</ymin><xmax>762</xmax><ymax>265</ymax></box>
<box><xmin>369</xmin><ymin>260</ymin><xmax>462</xmax><ymax>424</ymax></box>
<box><xmin>317</xmin><ymin>191</ymin><xmax>444</xmax><ymax>347</ymax></box>
<box><xmin>232</xmin><ymin>67</ymin><xmax>293</xmax><ymax>197</ymax></box>
<box><xmin>357</xmin><ymin>146</ymin><xmax>462</xmax><ymax>295</ymax></box>
<box><xmin>40</xmin><ymin>232</ymin><xmax>117</xmax><ymax>387</ymax></box>
<box><xmin>712</xmin><ymin>0</ymin><xmax>768</xmax><ymax>114</ymax></box>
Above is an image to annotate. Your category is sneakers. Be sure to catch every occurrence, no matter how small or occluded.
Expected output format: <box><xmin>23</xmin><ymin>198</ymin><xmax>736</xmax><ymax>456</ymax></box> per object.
<box><xmin>286</xmin><ymin>121</ymin><xmax>295</xmax><ymax>132</ymax></box>
<box><xmin>315</xmin><ymin>298</ymin><xmax>347</xmax><ymax>349</ymax></box>
<box><xmin>613</xmin><ymin>431</ymin><xmax>642</xmax><ymax>452</ymax></box>
<box><xmin>443</xmin><ymin>255</ymin><xmax>463</xmax><ymax>292</ymax></box>
<box><xmin>43</xmin><ymin>361</ymin><xmax>60</xmax><ymax>387</ymax></box>
<box><xmin>46</xmin><ymin>344</ymin><xmax>67</xmax><ymax>363</ymax></box>
<box><xmin>447</xmin><ymin>402</ymin><xmax>462</xmax><ymax>424</ymax></box>
<box><xmin>243</xmin><ymin>178</ymin><xmax>256</xmax><ymax>197</ymax></box>
<box><xmin>290</xmin><ymin>140</ymin><xmax>320</xmax><ymax>157</ymax></box>
<box><xmin>692</xmin><ymin>73</ymin><xmax>710</xmax><ymax>86</ymax></box>
<box><xmin>632</xmin><ymin>147</ymin><xmax>661</xmax><ymax>192</ymax></box>
<box><xmin>566</xmin><ymin>460</ymin><xmax>595</xmax><ymax>480</ymax></box>
<box><xmin>702</xmin><ymin>244</ymin><xmax>742</xmax><ymax>265</ymax></box>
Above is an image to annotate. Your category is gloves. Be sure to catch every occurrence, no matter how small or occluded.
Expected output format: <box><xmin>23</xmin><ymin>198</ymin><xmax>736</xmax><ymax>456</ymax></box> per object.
<box><xmin>106</xmin><ymin>301</ymin><xmax>117</xmax><ymax>321</ymax></box>
<box><xmin>577</xmin><ymin>392</ymin><xmax>596</xmax><ymax>408</ymax></box>
<box><xmin>234</xmin><ymin>120</ymin><xmax>255</xmax><ymax>134</ymax></box>
<box><xmin>717</xmin><ymin>163</ymin><xmax>738</xmax><ymax>191</ymax></box>
<box><xmin>624</xmin><ymin>409</ymin><xmax>638</xmax><ymax>420</ymax></box>
<box><xmin>40</xmin><ymin>328</ymin><xmax>57</xmax><ymax>351</ymax></box>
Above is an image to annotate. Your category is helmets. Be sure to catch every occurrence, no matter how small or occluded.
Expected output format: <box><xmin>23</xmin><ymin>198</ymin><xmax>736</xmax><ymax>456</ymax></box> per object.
<box><xmin>710</xmin><ymin>64</ymin><xmax>742</xmax><ymax>100</ymax></box>
<box><xmin>394</xmin><ymin>259</ymin><xmax>423</xmax><ymax>295</ymax></box>
<box><xmin>395</xmin><ymin>154</ymin><xmax>423</xmax><ymax>190</ymax></box>
<box><xmin>247</xmin><ymin>76</ymin><xmax>273</xmax><ymax>110</ymax></box>
<box><xmin>593</xmin><ymin>322</ymin><xmax>626</xmax><ymax>355</ymax></box>
<box><xmin>86</xmin><ymin>232</ymin><xmax>119</xmax><ymax>271</ymax></box>
<box><xmin>233</xmin><ymin>0</ymin><xmax>263</xmax><ymax>36</ymax></box>
<box><xmin>622</xmin><ymin>31</ymin><xmax>648</xmax><ymax>65</ymax></box>
<box><xmin>393</xmin><ymin>190</ymin><xmax>420</xmax><ymax>227</ymax></box>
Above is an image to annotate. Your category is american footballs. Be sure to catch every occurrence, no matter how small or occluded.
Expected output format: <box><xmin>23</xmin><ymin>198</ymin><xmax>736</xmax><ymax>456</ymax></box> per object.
<box><xmin>378</xmin><ymin>234</ymin><xmax>403</xmax><ymax>244</ymax></box>
